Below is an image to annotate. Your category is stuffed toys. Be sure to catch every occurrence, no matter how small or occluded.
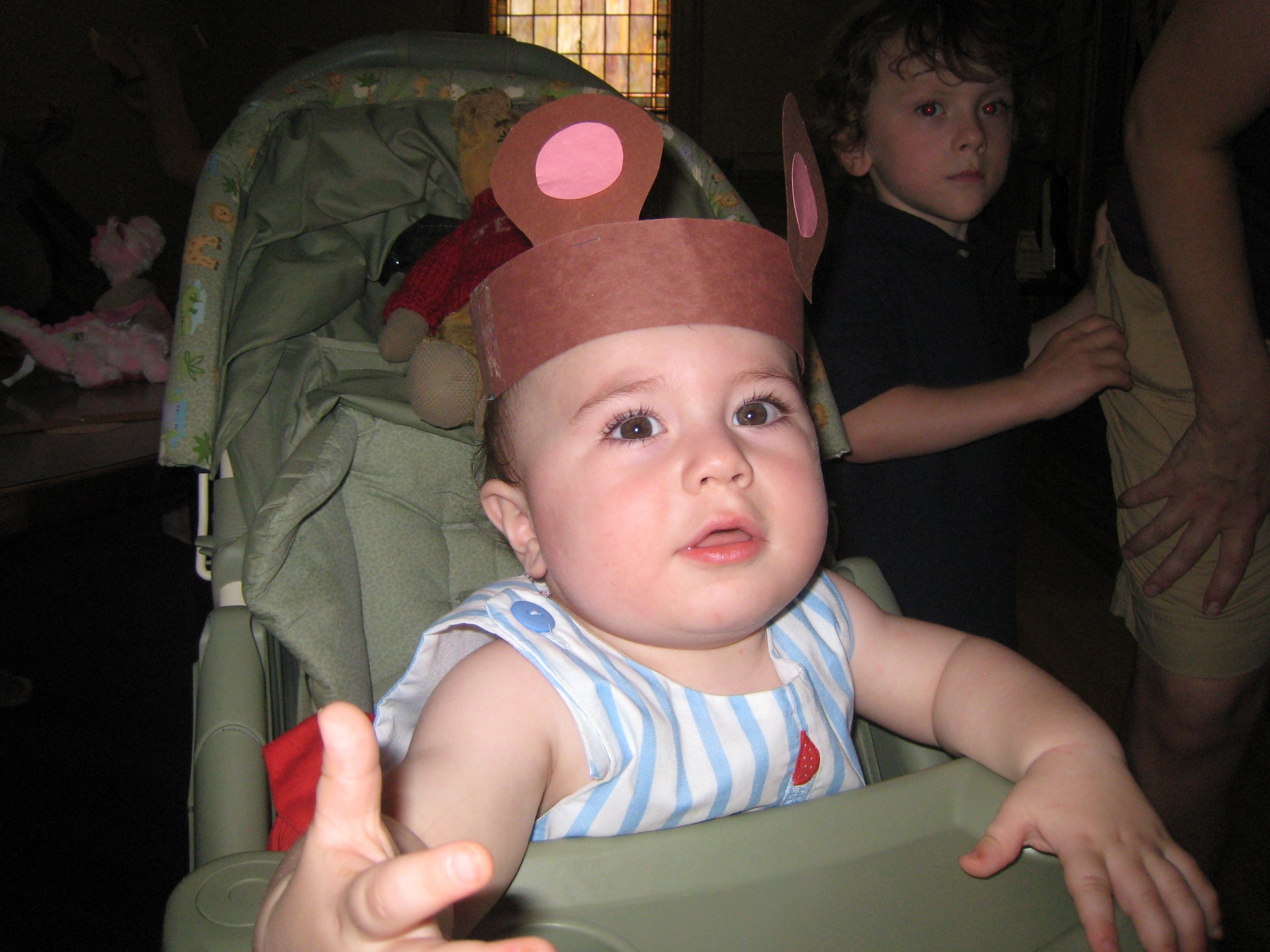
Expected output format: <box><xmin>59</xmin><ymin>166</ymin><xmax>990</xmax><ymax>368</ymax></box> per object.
<box><xmin>376</xmin><ymin>87</ymin><xmax>534</xmax><ymax>428</ymax></box>
<box><xmin>0</xmin><ymin>215</ymin><xmax>174</xmax><ymax>390</ymax></box>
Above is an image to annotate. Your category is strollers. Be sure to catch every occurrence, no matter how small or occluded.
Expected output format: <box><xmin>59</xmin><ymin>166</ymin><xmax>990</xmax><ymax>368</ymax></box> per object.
<box><xmin>158</xmin><ymin>29</ymin><xmax>1167</xmax><ymax>952</ymax></box>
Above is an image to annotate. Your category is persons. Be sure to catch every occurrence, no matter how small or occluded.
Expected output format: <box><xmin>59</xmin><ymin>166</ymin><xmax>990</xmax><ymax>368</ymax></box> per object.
<box><xmin>1090</xmin><ymin>0</ymin><xmax>1270</xmax><ymax>880</ymax></box>
<box><xmin>787</xmin><ymin>0</ymin><xmax>1133</xmax><ymax>735</ymax></box>
<box><xmin>251</xmin><ymin>93</ymin><xmax>1223</xmax><ymax>952</ymax></box>
